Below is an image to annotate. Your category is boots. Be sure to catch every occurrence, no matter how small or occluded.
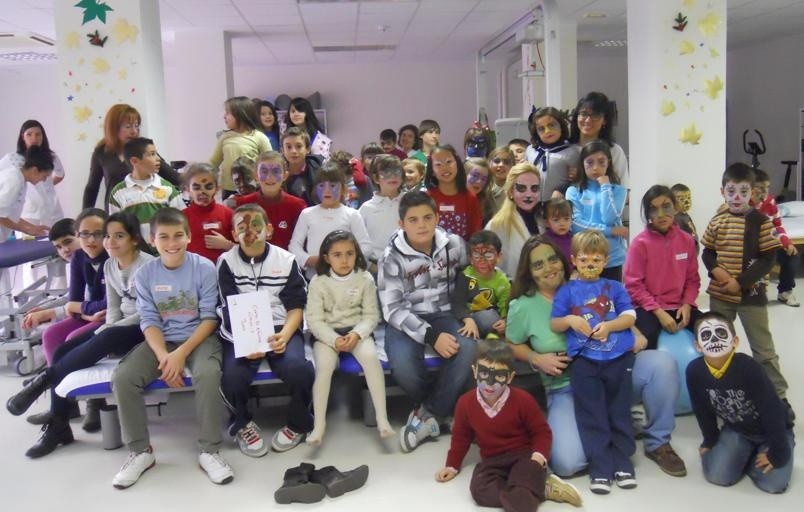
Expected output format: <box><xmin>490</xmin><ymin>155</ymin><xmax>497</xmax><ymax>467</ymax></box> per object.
<box><xmin>7</xmin><ymin>371</ymin><xmax>106</xmax><ymax>458</ymax></box>
<box><xmin>274</xmin><ymin>463</ymin><xmax>369</xmax><ymax>503</ymax></box>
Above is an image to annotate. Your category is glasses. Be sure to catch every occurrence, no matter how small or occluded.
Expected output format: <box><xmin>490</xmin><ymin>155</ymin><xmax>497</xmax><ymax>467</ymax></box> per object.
<box><xmin>579</xmin><ymin>110</ymin><xmax>601</xmax><ymax>121</ymax></box>
<box><xmin>77</xmin><ymin>230</ymin><xmax>104</xmax><ymax>239</ymax></box>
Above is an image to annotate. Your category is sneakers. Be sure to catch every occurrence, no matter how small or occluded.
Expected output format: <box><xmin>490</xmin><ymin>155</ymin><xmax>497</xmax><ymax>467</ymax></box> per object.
<box><xmin>777</xmin><ymin>289</ymin><xmax>800</xmax><ymax>306</ymax></box>
<box><xmin>401</xmin><ymin>407</ymin><xmax>439</xmax><ymax>452</ymax></box>
<box><xmin>645</xmin><ymin>444</ymin><xmax>686</xmax><ymax>476</ymax></box>
<box><xmin>234</xmin><ymin>422</ymin><xmax>268</xmax><ymax>457</ymax></box>
<box><xmin>113</xmin><ymin>447</ymin><xmax>156</xmax><ymax>487</ymax></box>
<box><xmin>614</xmin><ymin>471</ymin><xmax>637</xmax><ymax>488</ymax></box>
<box><xmin>589</xmin><ymin>478</ymin><xmax>611</xmax><ymax>492</ymax></box>
<box><xmin>272</xmin><ymin>427</ymin><xmax>304</xmax><ymax>453</ymax></box>
<box><xmin>544</xmin><ymin>470</ymin><xmax>582</xmax><ymax>508</ymax></box>
<box><xmin>200</xmin><ymin>452</ymin><xmax>233</xmax><ymax>485</ymax></box>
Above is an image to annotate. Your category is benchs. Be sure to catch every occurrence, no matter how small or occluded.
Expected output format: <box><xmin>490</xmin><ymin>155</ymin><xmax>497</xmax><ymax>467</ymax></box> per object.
<box><xmin>54</xmin><ymin>344</ymin><xmax>597</xmax><ymax>450</ymax></box>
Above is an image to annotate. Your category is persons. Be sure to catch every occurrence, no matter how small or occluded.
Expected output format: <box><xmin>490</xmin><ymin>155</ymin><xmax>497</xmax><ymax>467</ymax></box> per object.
<box><xmin>2</xmin><ymin>91</ymin><xmax>803</xmax><ymax>495</ymax></box>
<box><xmin>433</xmin><ymin>339</ymin><xmax>583</xmax><ymax>512</ymax></box>
